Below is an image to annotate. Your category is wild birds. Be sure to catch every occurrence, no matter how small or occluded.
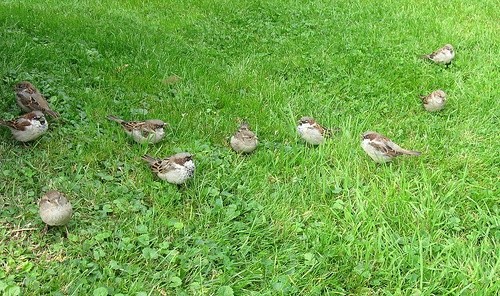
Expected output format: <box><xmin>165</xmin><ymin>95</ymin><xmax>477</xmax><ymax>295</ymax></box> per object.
<box><xmin>295</xmin><ymin>116</ymin><xmax>336</xmax><ymax>148</ymax></box>
<box><xmin>420</xmin><ymin>89</ymin><xmax>449</xmax><ymax>111</ymax></box>
<box><xmin>2</xmin><ymin>111</ymin><xmax>48</xmax><ymax>143</ymax></box>
<box><xmin>226</xmin><ymin>122</ymin><xmax>260</xmax><ymax>153</ymax></box>
<box><xmin>107</xmin><ymin>114</ymin><xmax>167</xmax><ymax>145</ymax></box>
<box><xmin>35</xmin><ymin>191</ymin><xmax>74</xmax><ymax>237</ymax></box>
<box><xmin>13</xmin><ymin>81</ymin><xmax>59</xmax><ymax>122</ymax></box>
<box><xmin>361</xmin><ymin>132</ymin><xmax>421</xmax><ymax>167</ymax></box>
<box><xmin>425</xmin><ymin>44</ymin><xmax>455</xmax><ymax>68</ymax></box>
<box><xmin>141</xmin><ymin>150</ymin><xmax>197</xmax><ymax>186</ymax></box>
